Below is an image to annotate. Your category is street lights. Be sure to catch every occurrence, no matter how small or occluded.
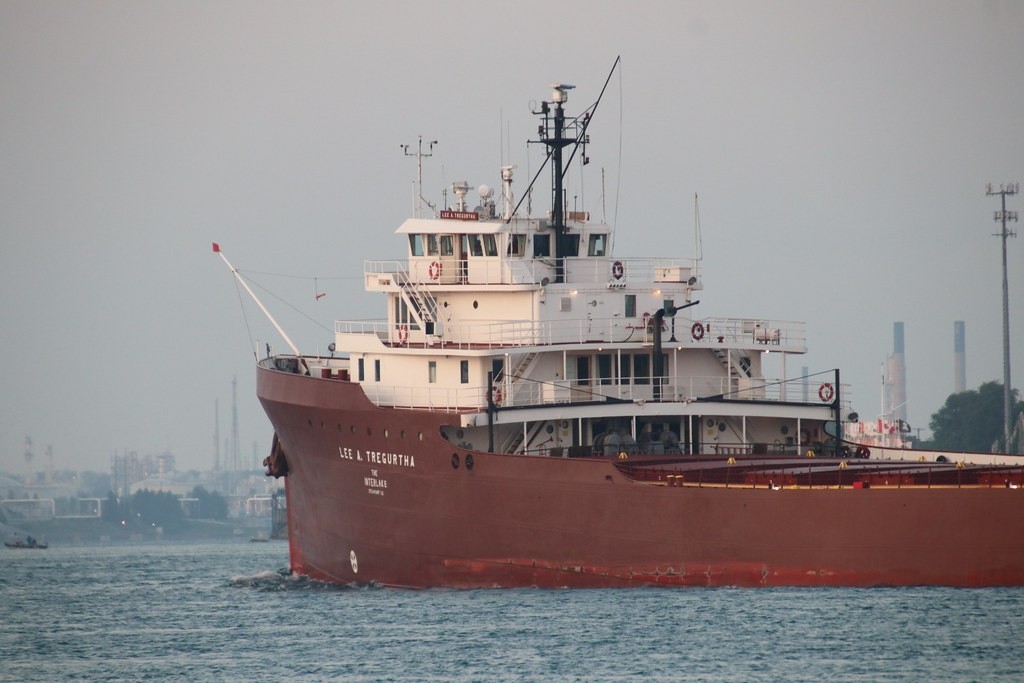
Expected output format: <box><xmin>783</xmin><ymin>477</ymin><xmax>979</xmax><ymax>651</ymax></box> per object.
<box><xmin>986</xmin><ymin>182</ymin><xmax>1020</xmax><ymax>453</ymax></box>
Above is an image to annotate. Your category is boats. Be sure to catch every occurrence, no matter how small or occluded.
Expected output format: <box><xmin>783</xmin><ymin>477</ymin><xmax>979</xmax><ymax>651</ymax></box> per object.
<box><xmin>212</xmin><ymin>55</ymin><xmax>1024</xmax><ymax>586</ymax></box>
<box><xmin>4</xmin><ymin>542</ymin><xmax>48</xmax><ymax>549</ymax></box>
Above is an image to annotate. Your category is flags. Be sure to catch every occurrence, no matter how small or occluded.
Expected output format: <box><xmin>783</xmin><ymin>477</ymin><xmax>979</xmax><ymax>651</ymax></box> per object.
<box><xmin>859</xmin><ymin>418</ymin><xmax>911</xmax><ymax>435</ymax></box>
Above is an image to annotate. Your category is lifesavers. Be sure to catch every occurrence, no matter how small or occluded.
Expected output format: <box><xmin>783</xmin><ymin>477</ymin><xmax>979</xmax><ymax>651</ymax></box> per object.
<box><xmin>613</xmin><ymin>261</ymin><xmax>623</xmax><ymax>279</ymax></box>
<box><xmin>399</xmin><ymin>326</ymin><xmax>408</xmax><ymax>344</ymax></box>
<box><xmin>795</xmin><ymin>427</ymin><xmax>810</xmax><ymax>446</ymax></box>
<box><xmin>428</xmin><ymin>262</ymin><xmax>443</xmax><ymax>279</ymax></box>
<box><xmin>691</xmin><ymin>323</ymin><xmax>704</xmax><ymax>340</ymax></box>
<box><xmin>819</xmin><ymin>383</ymin><xmax>834</xmax><ymax>402</ymax></box>
<box><xmin>485</xmin><ymin>387</ymin><xmax>501</xmax><ymax>405</ymax></box>
